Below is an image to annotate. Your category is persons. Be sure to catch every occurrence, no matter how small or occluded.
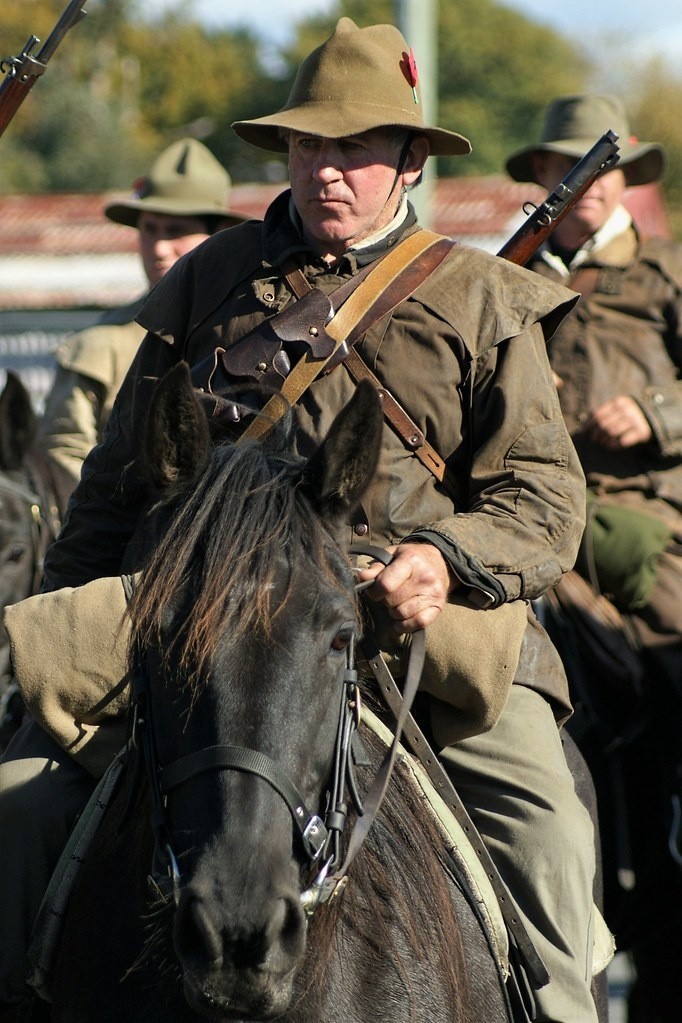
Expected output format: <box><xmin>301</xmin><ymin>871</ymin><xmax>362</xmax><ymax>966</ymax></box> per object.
<box><xmin>1</xmin><ymin>16</ymin><xmax>616</xmax><ymax>1023</ymax></box>
<box><xmin>36</xmin><ymin>139</ymin><xmax>259</xmax><ymax>540</ymax></box>
<box><xmin>505</xmin><ymin>94</ymin><xmax>682</xmax><ymax>712</ymax></box>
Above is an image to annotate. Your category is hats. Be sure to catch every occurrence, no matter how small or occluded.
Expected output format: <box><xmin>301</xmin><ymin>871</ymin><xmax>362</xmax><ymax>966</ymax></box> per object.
<box><xmin>503</xmin><ymin>96</ymin><xmax>668</xmax><ymax>186</ymax></box>
<box><xmin>228</xmin><ymin>16</ymin><xmax>473</xmax><ymax>157</ymax></box>
<box><xmin>103</xmin><ymin>137</ymin><xmax>255</xmax><ymax>230</ymax></box>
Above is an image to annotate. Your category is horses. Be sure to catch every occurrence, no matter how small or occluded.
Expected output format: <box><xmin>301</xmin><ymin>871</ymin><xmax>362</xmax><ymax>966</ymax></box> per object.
<box><xmin>1</xmin><ymin>358</ymin><xmax>609</xmax><ymax>1023</ymax></box>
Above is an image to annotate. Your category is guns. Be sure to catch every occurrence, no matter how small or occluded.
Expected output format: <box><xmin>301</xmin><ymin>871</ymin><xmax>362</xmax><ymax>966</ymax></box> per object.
<box><xmin>1</xmin><ymin>0</ymin><xmax>90</xmax><ymax>137</ymax></box>
<box><xmin>494</xmin><ymin>128</ymin><xmax>624</xmax><ymax>266</ymax></box>
